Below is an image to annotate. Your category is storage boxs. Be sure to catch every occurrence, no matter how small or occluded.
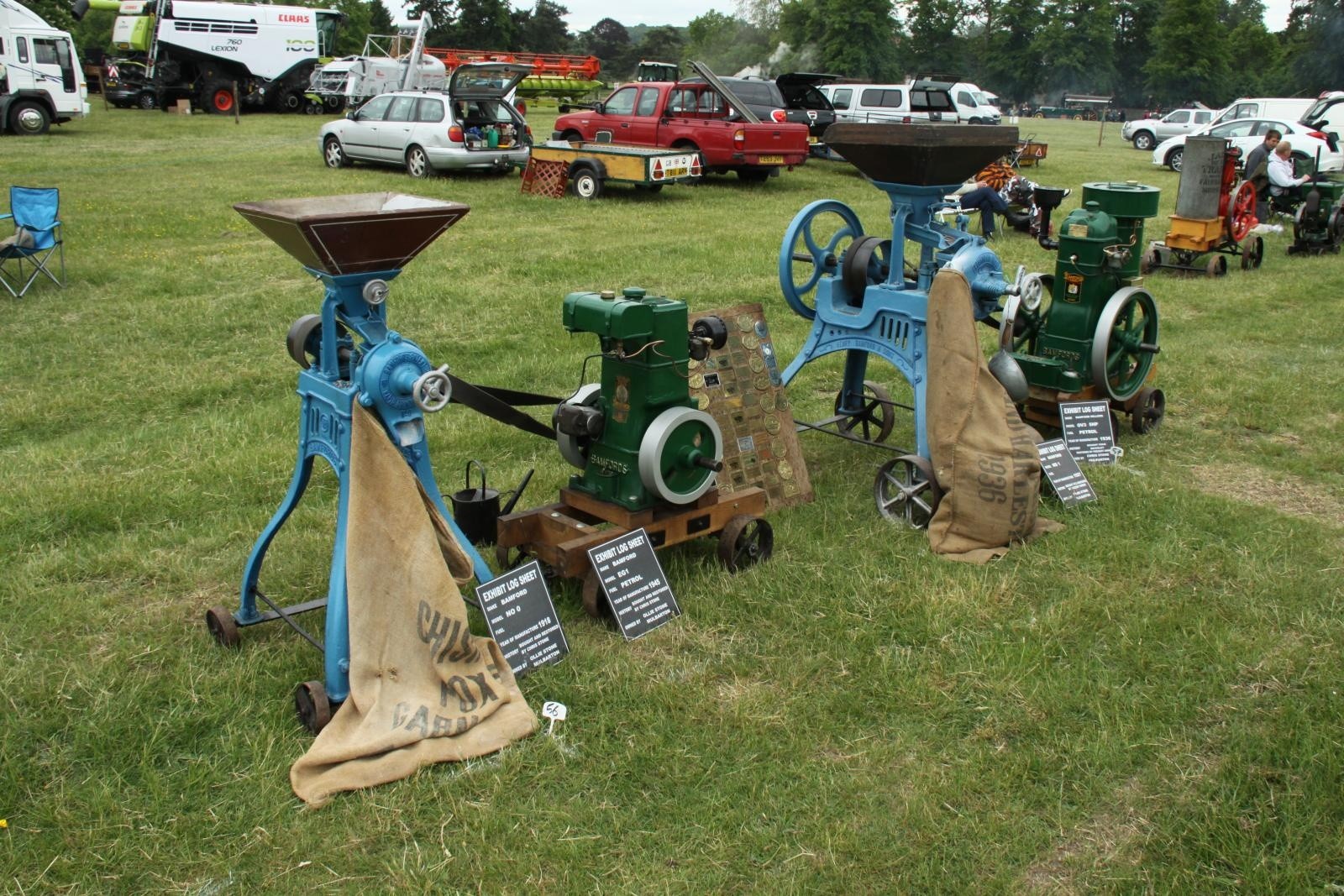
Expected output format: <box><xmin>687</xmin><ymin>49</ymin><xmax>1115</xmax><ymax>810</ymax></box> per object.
<box><xmin>168</xmin><ymin>99</ymin><xmax>191</xmax><ymax>115</ymax></box>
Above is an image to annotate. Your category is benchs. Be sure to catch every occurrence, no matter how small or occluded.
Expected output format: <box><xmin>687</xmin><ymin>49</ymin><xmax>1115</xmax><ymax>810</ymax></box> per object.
<box><xmin>935</xmin><ymin>195</ymin><xmax>1025</xmax><ymax>242</ymax></box>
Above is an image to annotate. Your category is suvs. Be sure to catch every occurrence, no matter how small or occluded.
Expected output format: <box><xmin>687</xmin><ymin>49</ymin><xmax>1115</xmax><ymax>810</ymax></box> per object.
<box><xmin>316</xmin><ymin>61</ymin><xmax>535</xmax><ymax>178</ymax></box>
<box><xmin>628</xmin><ymin>72</ymin><xmax>843</xmax><ymax>150</ymax></box>
<box><xmin>1122</xmin><ymin>107</ymin><xmax>1217</xmax><ymax>150</ymax></box>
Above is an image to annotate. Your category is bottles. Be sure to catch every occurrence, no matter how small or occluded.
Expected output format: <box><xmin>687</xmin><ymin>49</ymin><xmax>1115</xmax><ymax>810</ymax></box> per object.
<box><xmin>488</xmin><ymin>128</ymin><xmax>498</xmax><ymax>148</ymax></box>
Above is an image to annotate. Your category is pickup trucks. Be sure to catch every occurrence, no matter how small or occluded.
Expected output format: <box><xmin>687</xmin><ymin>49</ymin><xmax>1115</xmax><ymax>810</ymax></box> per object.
<box><xmin>549</xmin><ymin>70</ymin><xmax>810</xmax><ymax>183</ymax></box>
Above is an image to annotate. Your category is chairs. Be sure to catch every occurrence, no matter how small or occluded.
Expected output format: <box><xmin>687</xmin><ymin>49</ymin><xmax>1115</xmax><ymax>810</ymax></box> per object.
<box><xmin>0</xmin><ymin>186</ymin><xmax>66</xmax><ymax>298</ymax></box>
<box><xmin>1006</xmin><ymin>133</ymin><xmax>1038</xmax><ymax>169</ymax></box>
<box><xmin>1258</xmin><ymin>125</ymin><xmax>1269</xmax><ymax>136</ymax></box>
<box><xmin>1259</xmin><ymin>161</ymin><xmax>1300</xmax><ymax>222</ymax></box>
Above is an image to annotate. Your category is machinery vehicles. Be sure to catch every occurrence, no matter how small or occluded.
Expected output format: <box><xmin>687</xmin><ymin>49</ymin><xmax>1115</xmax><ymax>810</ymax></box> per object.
<box><xmin>301</xmin><ymin>11</ymin><xmax>605</xmax><ymax>115</ymax></box>
<box><xmin>71</xmin><ymin>0</ymin><xmax>349</xmax><ymax>115</ymax></box>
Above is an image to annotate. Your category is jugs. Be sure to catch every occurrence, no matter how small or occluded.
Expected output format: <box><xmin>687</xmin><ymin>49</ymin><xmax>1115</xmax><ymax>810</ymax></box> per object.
<box><xmin>440</xmin><ymin>460</ymin><xmax>535</xmax><ymax>549</ymax></box>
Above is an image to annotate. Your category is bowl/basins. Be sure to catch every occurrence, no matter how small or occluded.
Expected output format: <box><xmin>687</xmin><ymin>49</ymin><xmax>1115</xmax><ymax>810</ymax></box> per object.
<box><xmin>988</xmin><ymin>350</ymin><xmax>1029</xmax><ymax>401</ymax></box>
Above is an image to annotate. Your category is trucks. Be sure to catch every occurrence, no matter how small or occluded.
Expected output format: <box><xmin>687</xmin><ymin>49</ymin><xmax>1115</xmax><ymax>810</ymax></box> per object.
<box><xmin>0</xmin><ymin>0</ymin><xmax>90</xmax><ymax>136</ymax></box>
<box><xmin>1031</xmin><ymin>93</ymin><xmax>1125</xmax><ymax>123</ymax></box>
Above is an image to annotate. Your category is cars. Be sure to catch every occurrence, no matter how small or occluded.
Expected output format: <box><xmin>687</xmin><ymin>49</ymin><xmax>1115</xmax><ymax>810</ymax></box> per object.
<box><xmin>1151</xmin><ymin>116</ymin><xmax>1344</xmax><ymax>175</ymax></box>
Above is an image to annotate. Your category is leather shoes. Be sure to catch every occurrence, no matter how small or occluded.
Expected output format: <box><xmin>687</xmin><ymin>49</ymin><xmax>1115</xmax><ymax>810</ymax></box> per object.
<box><xmin>1008</xmin><ymin>214</ymin><xmax>1033</xmax><ymax>226</ymax></box>
<box><xmin>984</xmin><ymin>234</ymin><xmax>992</xmax><ymax>242</ymax></box>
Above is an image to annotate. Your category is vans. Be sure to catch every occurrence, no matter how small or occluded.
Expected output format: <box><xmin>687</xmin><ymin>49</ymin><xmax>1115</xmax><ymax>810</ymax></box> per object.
<box><xmin>809</xmin><ymin>79</ymin><xmax>1003</xmax><ymax>131</ymax></box>
<box><xmin>1191</xmin><ymin>91</ymin><xmax>1344</xmax><ymax>150</ymax></box>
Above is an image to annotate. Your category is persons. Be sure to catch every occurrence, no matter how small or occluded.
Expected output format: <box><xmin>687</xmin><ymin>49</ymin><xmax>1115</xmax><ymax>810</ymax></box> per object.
<box><xmin>944</xmin><ymin>177</ymin><xmax>1032</xmax><ymax>243</ymax></box>
<box><xmin>1005</xmin><ymin>101</ymin><xmax>1030</xmax><ymax>117</ymax></box>
<box><xmin>1242</xmin><ymin>129</ymin><xmax>1311</xmax><ymax>224</ymax></box>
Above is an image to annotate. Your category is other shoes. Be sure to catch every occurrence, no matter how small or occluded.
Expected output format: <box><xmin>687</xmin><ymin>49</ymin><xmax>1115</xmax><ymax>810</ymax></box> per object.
<box><xmin>1062</xmin><ymin>188</ymin><xmax>1071</xmax><ymax>198</ymax></box>
